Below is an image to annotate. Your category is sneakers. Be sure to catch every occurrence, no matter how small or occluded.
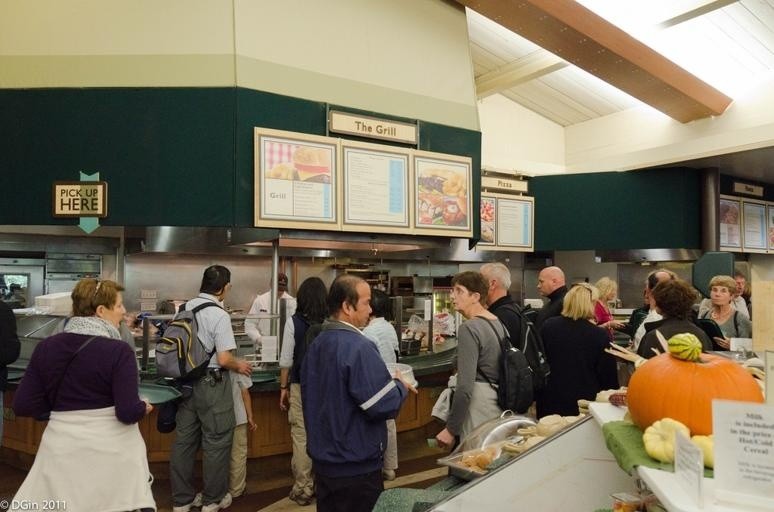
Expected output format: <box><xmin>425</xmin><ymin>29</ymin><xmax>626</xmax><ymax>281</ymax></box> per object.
<box><xmin>290</xmin><ymin>490</ymin><xmax>311</xmax><ymax>508</ymax></box>
<box><xmin>172</xmin><ymin>493</ymin><xmax>203</xmax><ymax>511</ymax></box>
<box><xmin>204</xmin><ymin>492</ymin><xmax>233</xmax><ymax>512</ymax></box>
<box><xmin>381</xmin><ymin>468</ymin><xmax>395</xmax><ymax>480</ymax></box>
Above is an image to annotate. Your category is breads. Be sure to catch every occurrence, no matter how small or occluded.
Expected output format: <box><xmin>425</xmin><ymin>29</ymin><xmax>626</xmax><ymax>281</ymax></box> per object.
<box><xmin>264</xmin><ymin>148</ymin><xmax>330</xmax><ymax>184</ymax></box>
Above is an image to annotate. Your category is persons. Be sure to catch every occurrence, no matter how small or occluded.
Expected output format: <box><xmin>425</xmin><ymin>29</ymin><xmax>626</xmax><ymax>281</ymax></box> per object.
<box><xmin>435</xmin><ymin>264</ymin><xmax>752</xmax><ymax>453</ymax></box>
<box><xmin>5</xmin><ymin>275</ymin><xmax>159</xmax><ymax>511</ymax></box>
<box><xmin>154</xmin><ymin>264</ymin><xmax>252</xmax><ymax>511</ymax></box>
<box><xmin>0</xmin><ymin>299</ymin><xmax>23</xmax><ymax>474</ymax></box>
<box><xmin>280</xmin><ymin>274</ymin><xmax>418</xmax><ymax>512</ymax></box>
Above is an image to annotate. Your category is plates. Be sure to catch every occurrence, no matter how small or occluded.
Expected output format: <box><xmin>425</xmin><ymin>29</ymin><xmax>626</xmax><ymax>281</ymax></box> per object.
<box><xmin>720</xmin><ymin>201</ymin><xmax>739</xmax><ymax>224</ymax></box>
<box><xmin>34</xmin><ymin>291</ymin><xmax>73</xmax><ymax>313</ymax></box>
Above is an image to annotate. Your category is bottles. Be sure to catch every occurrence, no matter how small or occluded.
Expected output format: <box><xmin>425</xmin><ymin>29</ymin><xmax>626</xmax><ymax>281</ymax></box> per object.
<box><xmin>616</xmin><ymin>298</ymin><xmax>622</xmax><ymax>308</ymax></box>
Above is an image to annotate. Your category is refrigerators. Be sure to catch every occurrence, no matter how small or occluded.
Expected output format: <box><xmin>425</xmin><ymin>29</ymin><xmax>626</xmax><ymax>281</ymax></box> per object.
<box><xmin>413</xmin><ymin>275</ymin><xmax>462</xmax><ymax>336</ymax></box>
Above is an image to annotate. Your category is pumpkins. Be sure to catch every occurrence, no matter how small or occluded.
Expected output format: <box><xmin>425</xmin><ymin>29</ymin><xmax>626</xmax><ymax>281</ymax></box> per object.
<box><xmin>627</xmin><ymin>351</ymin><xmax>765</xmax><ymax>436</ymax></box>
<box><xmin>668</xmin><ymin>332</ymin><xmax>700</xmax><ymax>359</ymax></box>
<box><xmin>642</xmin><ymin>417</ymin><xmax>690</xmax><ymax>464</ymax></box>
<box><xmin>689</xmin><ymin>436</ymin><xmax>715</xmax><ymax>468</ymax></box>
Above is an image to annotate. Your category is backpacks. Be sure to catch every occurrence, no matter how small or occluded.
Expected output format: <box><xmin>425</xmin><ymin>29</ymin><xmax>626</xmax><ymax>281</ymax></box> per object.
<box><xmin>467</xmin><ymin>314</ymin><xmax>534</xmax><ymax>414</ymax></box>
<box><xmin>156</xmin><ymin>303</ymin><xmax>225</xmax><ymax>381</ymax></box>
<box><xmin>494</xmin><ymin>302</ymin><xmax>552</xmax><ymax>400</ymax></box>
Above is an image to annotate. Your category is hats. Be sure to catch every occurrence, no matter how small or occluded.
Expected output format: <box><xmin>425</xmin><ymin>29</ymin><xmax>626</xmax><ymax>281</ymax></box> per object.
<box><xmin>275</xmin><ymin>274</ymin><xmax>289</xmax><ymax>293</ymax></box>
